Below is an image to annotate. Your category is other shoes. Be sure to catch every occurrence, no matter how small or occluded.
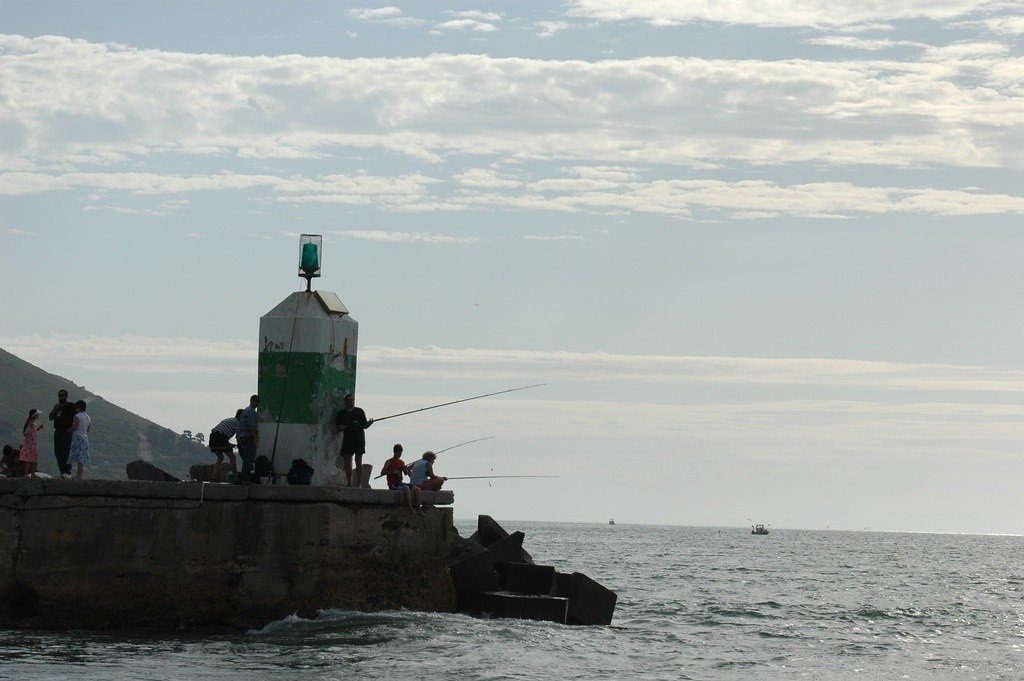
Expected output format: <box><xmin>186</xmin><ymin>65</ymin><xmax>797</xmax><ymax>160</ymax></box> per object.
<box><xmin>6</xmin><ymin>471</ymin><xmax>13</xmax><ymax>476</ymax></box>
<box><xmin>209</xmin><ymin>478</ymin><xmax>218</xmax><ymax>482</ymax></box>
<box><xmin>234</xmin><ymin>479</ymin><xmax>242</xmax><ymax>485</ymax></box>
<box><xmin>23</xmin><ymin>473</ymin><xmax>29</xmax><ymax>478</ymax></box>
<box><xmin>240</xmin><ymin>479</ymin><xmax>252</xmax><ymax>486</ymax></box>
<box><xmin>31</xmin><ymin>474</ymin><xmax>41</xmax><ymax>478</ymax></box>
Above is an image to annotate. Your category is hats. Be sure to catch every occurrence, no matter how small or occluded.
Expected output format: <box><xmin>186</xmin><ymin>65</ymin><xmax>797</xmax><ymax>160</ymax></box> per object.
<box><xmin>30</xmin><ymin>408</ymin><xmax>43</xmax><ymax>417</ymax></box>
<box><xmin>250</xmin><ymin>395</ymin><xmax>261</xmax><ymax>402</ymax></box>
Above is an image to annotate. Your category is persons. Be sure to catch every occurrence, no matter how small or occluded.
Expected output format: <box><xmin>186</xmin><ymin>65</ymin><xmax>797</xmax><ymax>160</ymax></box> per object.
<box><xmin>19</xmin><ymin>409</ymin><xmax>43</xmax><ymax>479</ymax></box>
<box><xmin>380</xmin><ymin>445</ymin><xmax>426</xmax><ymax>517</ymax></box>
<box><xmin>239</xmin><ymin>396</ymin><xmax>260</xmax><ymax>486</ymax></box>
<box><xmin>208</xmin><ymin>410</ymin><xmax>243</xmax><ymax>482</ymax></box>
<box><xmin>0</xmin><ymin>445</ymin><xmax>20</xmax><ymax>477</ymax></box>
<box><xmin>335</xmin><ymin>395</ymin><xmax>373</xmax><ymax>489</ymax></box>
<box><xmin>405</xmin><ymin>452</ymin><xmax>447</xmax><ymax>491</ymax></box>
<box><xmin>65</xmin><ymin>400</ymin><xmax>90</xmax><ymax>479</ymax></box>
<box><xmin>48</xmin><ymin>390</ymin><xmax>76</xmax><ymax>479</ymax></box>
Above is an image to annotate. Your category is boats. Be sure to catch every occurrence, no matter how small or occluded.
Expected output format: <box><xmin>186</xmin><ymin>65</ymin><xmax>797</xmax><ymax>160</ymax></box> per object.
<box><xmin>751</xmin><ymin>524</ymin><xmax>769</xmax><ymax>535</ymax></box>
<box><xmin>609</xmin><ymin>520</ymin><xmax>615</xmax><ymax>525</ymax></box>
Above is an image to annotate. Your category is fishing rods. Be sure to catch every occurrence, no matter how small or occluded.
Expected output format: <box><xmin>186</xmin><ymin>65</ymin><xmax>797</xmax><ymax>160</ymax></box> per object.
<box><xmin>374</xmin><ymin>437</ymin><xmax>495</xmax><ymax>479</ymax></box>
<box><xmin>345</xmin><ymin>383</ymin><xmax>547</xmax><ymax>429</ymax></box>
<box><xmin>447</xmin><ymin>475</ymin><xmax>560</xmax><ymax>480</ymax></box>
<box><xmin>267</xmin><ymin>283</ymin><xmax>302</xmax><ymax>484</ymax></box>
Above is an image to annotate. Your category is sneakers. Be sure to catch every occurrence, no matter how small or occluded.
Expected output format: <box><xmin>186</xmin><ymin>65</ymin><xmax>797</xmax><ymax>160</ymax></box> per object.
<box><xmin>62</xmin><ymin>472</ymin><xmax>72</xmax><ymax>479</ymax></box>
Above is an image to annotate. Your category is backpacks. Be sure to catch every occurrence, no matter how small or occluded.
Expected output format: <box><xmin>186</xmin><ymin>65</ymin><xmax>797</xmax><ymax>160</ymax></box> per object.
<box><xmin>252</xmin><ymin>455</ymin><xmax>271</xmax><ymax>484</ymax></box>
<box><xmin>287</xmin><ymin>459</ymin><xmax>314</xmax><ymax>485</ymax></box>
